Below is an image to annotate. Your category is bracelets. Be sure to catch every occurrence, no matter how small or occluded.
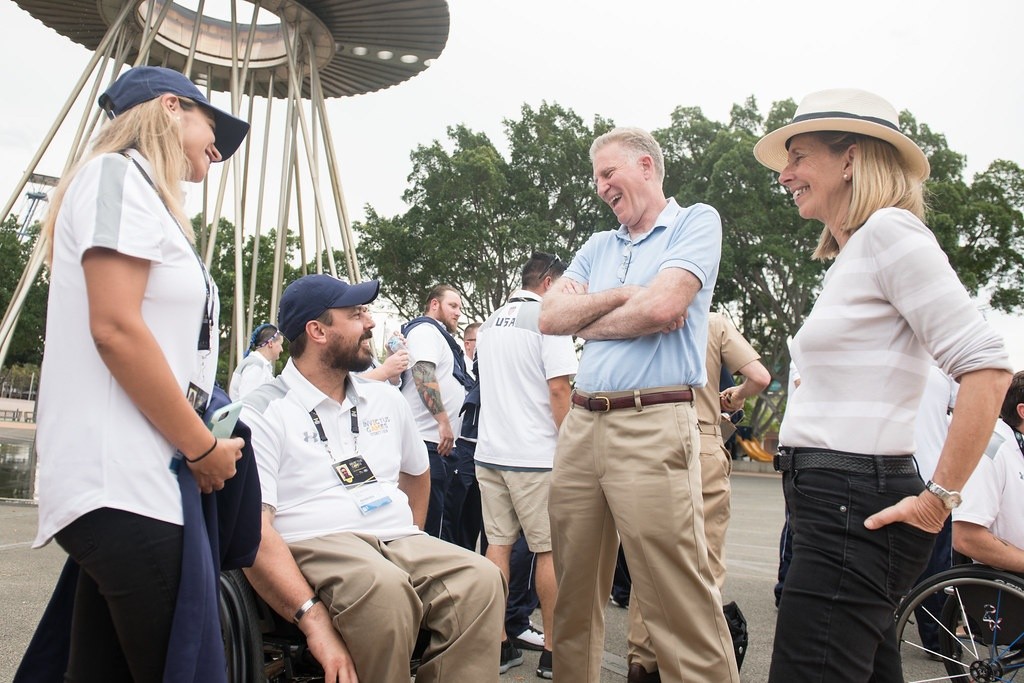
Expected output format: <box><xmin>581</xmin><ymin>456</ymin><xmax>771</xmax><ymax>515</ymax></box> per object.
<box><xmin>292</xmin><ymin>596</ymin><xmax>322</xmax><ymax>627</ymax></box>
<box><xmin>185</xmin><ymin>438</ymin><xmax>218</xmax><ymax>464</ymax></box>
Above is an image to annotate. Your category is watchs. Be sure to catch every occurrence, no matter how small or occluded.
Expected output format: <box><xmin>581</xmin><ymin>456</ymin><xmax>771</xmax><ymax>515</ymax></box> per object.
<box><xmin>923</xmin><ymin>480</ymin><xmax>963</xmax><ymax>509</ymax></box>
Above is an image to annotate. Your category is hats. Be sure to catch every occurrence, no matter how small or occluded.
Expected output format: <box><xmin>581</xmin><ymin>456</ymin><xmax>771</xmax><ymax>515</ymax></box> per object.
<box><xmin>753</xmin><ymin>89</ymin><xmax>930</xmax><ymax>183</ymax></box>
<box><xmin>278</xmin><ymin>274</ymin><xmax>380</xmax><ymax>342</ymax></box>
<box><xmin>98</xmin><ymin>66</ymin><xmax>251</xmax><ymax>163</ymax></box>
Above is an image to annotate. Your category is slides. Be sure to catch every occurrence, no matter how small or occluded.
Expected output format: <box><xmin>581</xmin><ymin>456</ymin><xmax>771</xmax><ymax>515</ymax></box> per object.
<box><xmin>736</xmin><ymin>434</ymin><xmax>773</xmax><ymax>462</ymax></box>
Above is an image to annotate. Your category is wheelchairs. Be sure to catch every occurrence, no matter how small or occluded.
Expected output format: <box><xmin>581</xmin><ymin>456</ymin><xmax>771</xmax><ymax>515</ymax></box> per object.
<box><xmin>892</xmin><ymin>562</ymin><xmax>1024</xmax><ymax>683</ymax></box>
<box><xmin>217</xmin><ymin>565</ymin><xmax>428</xmax><ymax>683</ymax></box>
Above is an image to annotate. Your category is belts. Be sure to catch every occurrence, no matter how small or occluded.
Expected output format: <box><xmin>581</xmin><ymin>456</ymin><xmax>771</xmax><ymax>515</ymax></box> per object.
<box><xmin>773</xmin><ymin>452</ymin><xmax>918</xmax><ymax>475</ymax></box>
<box><xmin>700</xmin><ymin>425</ymin><xmax>722</xmax><ymax>435</ymax></box>
<box><xmin>572</xmin><ymin>390</ymin><xmax>692</xmax><ymax>413</ymax></box>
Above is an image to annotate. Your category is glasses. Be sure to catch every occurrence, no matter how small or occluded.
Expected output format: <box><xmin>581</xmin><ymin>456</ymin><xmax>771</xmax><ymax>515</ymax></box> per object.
<box><xmin>539</xmin><ymin>254</ymin><xmax>561</xmax><ymax>279</ymax></box>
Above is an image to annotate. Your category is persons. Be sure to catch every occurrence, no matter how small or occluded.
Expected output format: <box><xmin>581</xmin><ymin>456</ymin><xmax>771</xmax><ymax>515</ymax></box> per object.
<box><xmin>341</xmin><ymin>468</ymin><xmax>351</xmax><ymax>479</ymax></box>
<box><xmin>912</xmin><ymin>380</ymin><xmax>967</xmax><ymax>663</ymax></box>
<box><xmin>538</xmin><ymin>128</ymin><xmax>739</xmax><ymax>683</ymax></box>
<box><xmin>952</xmin><ymin>370</ymin><xmax>1023</xmax><ymax>657</ymax></box>
<box><xmin>626</xmin><ymin>313</ymin><xmax>771</xmax><ymax>683</ymax></box>
<box><xmin>752</xmin><ymin>91</ymin><xmax>1014</xmax><ymax>682</ymax></box>
<box><xmin>10</xmin><ymin>66</ymin><xmax>251</xmax><ymax>683</ymax></box>
<box><xmin>189</xmin><ymin>392</ymin><xmax>194</xmax><ymax>406</ymax></box>
<box><xmin>228</xmin><ymin>248</ymin><xmax>632</xmax><ymax>683</ymax></box>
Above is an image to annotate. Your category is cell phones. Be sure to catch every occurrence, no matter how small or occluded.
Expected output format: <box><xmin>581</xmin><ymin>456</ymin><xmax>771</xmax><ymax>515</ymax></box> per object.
<box><xmin>207</xmin><ymin>402</ymin><xmax>242</xmax><ymax>440</ymax></box>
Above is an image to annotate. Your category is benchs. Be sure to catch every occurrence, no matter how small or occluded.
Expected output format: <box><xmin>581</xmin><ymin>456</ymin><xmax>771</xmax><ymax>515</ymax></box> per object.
<box><xmin>25</xmin><ymin>412</ymin><xmax>34</xmax><ymax>422</ymax></box>
<box><xmin>0</xmin><ymin>410</ymin><xmax>22</xmax><ymax>421</ymax></box>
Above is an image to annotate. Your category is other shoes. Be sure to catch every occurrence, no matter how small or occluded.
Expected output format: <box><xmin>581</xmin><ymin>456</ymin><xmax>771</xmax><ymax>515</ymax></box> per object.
<box><xmin>929</xmin><ymin>651</ymin><xmax>959</xmax><ymax>662</ymax></box>
<box><xmin>627</xmin><ymin>662</ymin><xmax>661</xmax><ymax>683</ymax></box>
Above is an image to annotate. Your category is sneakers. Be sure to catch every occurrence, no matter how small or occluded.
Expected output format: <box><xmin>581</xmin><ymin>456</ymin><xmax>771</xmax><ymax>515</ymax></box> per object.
<box><xmin>513</xmin><ymin>620</ymin><xmax>544</xmax><ymax>650</ymax></box>
<box><xmin>500</xmin><ymin>637</ymin><xmax>523</xmax><ymax>673</ymax></box>
<box><xmin>536</xmin><ymin>648</ymin><xmax>552</xmax><ymax>678</ymax></box>
